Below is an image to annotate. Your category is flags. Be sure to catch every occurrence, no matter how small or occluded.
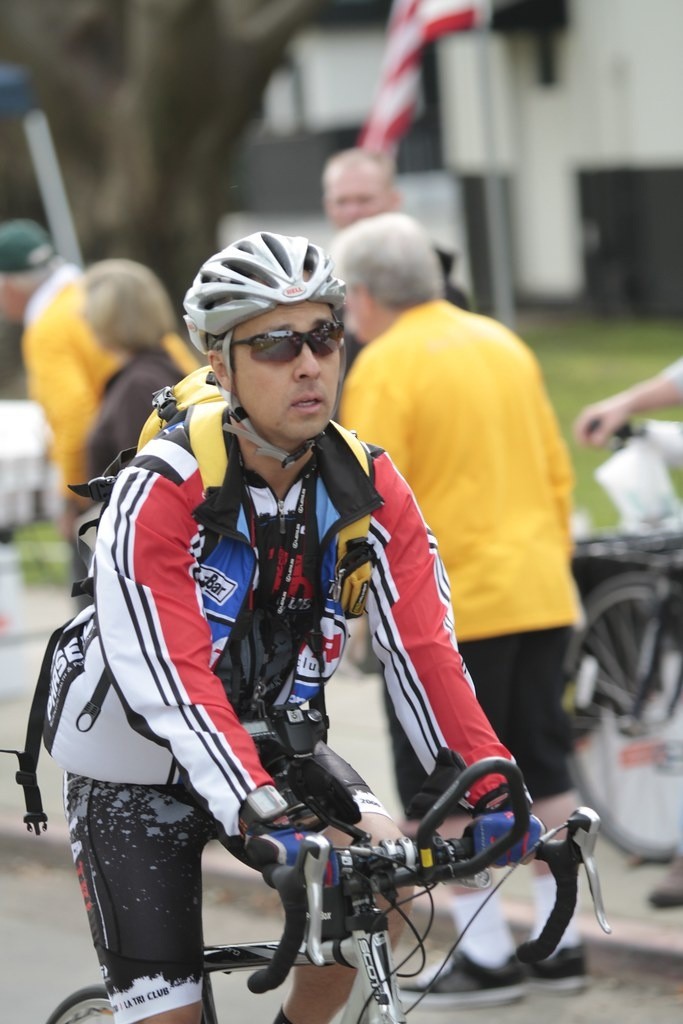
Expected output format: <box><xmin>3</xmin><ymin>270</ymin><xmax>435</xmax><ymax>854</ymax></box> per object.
<box><xmin>355</xmin><ymin>1</ymin><xmax>494</xmax><ymax>150</ymax></box>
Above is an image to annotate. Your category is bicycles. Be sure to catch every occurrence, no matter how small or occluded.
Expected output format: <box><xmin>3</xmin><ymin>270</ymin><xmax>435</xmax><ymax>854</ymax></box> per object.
<box><xmin>50</xmin><ymin>756</ymin><xmax>615</xmax><ymax>1023</ymax></box>
<box><xmin>560</xmin><ymin>420</ymin><xmax>683</xmax><ymax>866</ymax></box>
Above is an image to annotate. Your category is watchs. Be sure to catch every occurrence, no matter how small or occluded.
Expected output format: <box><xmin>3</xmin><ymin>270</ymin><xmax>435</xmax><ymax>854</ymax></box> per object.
<box><xmin>238</xmin><ymin>784</ymin><xmax>288</xmax><ymax>839</ymax></box>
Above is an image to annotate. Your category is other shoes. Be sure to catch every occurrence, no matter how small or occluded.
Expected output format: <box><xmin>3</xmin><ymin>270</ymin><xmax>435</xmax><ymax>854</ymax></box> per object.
<box><xmin>528</xmin><ymin>940</ymin><xmax>587</xmax><ymax>989</ymax></box>
<box><xmin>396</xmin><ymin>949</ymin><xmax>528</xmax><ymax>1008</ymax></box>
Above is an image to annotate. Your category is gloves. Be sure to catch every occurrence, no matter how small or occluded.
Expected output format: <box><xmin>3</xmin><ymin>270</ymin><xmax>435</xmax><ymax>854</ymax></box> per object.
<box><xmin>463</xmin><ymin>811</ymin><xmax>546</xmax><ymax>866</ymax></box>
<box><xmin>245</xmin><ymin>827</ymin><xmax>340</xmax><ymax>889</ymax></box>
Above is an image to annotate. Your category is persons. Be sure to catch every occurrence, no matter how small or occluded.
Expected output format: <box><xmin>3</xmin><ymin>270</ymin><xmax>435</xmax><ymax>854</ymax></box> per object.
<box><xmin>0</xmin><ymin>150</ymin><xmax>683</xmax><ymax>1024</ymax></box>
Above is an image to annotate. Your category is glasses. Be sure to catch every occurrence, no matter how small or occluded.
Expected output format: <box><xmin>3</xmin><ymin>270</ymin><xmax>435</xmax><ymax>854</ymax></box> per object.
<box><xmin>213</xmin><ymin>321</ymin><xmax>345</xmax><ymax>364</ymax></box>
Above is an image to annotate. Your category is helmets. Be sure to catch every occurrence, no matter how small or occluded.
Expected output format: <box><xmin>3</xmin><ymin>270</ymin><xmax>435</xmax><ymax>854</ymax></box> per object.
<box><xmin>182</xmin><ymin>230</ymin><xmax>346</xmax><ymax>356</ymax></box>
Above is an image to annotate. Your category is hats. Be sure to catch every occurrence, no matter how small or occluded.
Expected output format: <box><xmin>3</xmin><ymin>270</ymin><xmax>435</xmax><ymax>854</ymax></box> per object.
<box><xmin>0</xmin><ymin>219</ymin><xmax>57</xmax><ymax>271</ymax></box>
